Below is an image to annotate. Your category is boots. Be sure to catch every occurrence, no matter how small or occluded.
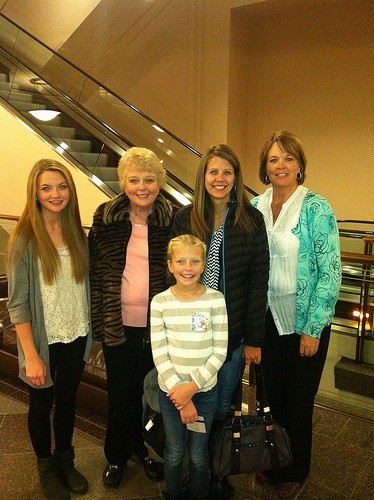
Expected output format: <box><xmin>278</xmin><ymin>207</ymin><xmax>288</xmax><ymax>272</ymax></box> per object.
<box><xmin>37</xmin><ymin>446</ymin><xmax>88</xmax><ymax>500</ymax></box>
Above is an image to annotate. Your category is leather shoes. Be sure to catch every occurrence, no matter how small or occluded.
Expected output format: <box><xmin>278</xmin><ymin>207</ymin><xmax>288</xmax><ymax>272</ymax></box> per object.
<box><xmin>102</xmin><ymin>450</ymin><xmax>164</xmax><ymax>488</ymax></box>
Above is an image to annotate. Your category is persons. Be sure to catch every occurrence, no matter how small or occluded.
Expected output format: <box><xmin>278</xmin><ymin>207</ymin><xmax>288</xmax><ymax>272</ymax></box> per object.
<box><xmin>165</xmin><ymin>145</ymin><xmax>271</xmax><ymax>500</ymax></box>
<box><xmin>248</xmin><ymin>133</ymin><xmax>341</xmax><ymax>500</ymax></box>
<box><xmin>151</xmin><ymin>235</ymin><xmax>228</xmax><ymax>500</ymax></box>
<box><xmin>88</xmin><ymin>147</ymin><xmax>188</xmax><ymax>485</ymax></box>
<box><xmin>8</xmin><ymin>160</ymin><xmax>92</xmax><ymax>500</ymax></box>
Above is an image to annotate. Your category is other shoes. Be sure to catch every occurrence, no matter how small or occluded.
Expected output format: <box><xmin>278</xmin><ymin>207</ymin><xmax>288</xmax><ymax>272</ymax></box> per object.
<box><xmin>184</xmin><ymin>475</ymin><xmax>234</xmax><ymax>500</ymax></box>
<box><xmin>254</xmin><ymin>472</ymin><xmax>300</xmax><ymax>499</ymax></box>
<box><xmin>212</xmin><ymin>358</ymin><xmax>293</xmax><ymax>477</ymax></box>
